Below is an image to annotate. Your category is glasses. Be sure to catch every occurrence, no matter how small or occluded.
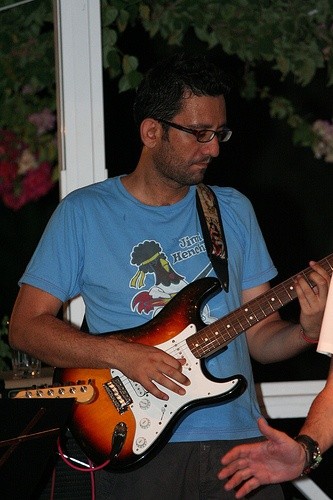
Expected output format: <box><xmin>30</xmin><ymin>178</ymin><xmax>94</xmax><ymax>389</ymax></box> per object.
<box><xmin>151</xmin><ymin>117</ymin><xmax>233</xmax><ymax>143</ymax></box>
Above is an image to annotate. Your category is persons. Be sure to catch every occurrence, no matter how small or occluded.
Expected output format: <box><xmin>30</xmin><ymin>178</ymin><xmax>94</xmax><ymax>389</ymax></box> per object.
<box><xmin>7</xmin><ymin>53</ymin><xmax>330</xmax><ymax>500</ymax></box>
<box><xmin>218</xmin><ymin>270</ymin><xmax>333</xmax><ymax>497</ymax></box>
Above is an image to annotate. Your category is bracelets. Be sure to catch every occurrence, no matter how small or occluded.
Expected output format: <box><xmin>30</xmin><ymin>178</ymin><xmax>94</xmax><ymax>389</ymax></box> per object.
<box><xmin>299</xmin><ymin>326</ymin><xmax>320</xmax><ymax>343</ymax></box>
<box><xmin>293</xmin><ymin>434</ymin><xmax>323</xmax><ymax>478</ymax></box>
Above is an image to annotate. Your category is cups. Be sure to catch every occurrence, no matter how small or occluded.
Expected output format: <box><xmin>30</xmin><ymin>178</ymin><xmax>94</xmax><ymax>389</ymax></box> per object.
<box><xmin>11</xmin><ymin>350</ymin><xmax>42</xmax><ymax>376</ymax></box>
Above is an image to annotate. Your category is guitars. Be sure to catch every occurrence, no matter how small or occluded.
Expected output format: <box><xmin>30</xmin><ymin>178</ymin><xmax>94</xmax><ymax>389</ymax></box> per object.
<box><xmin>0</xmin><ymin>379</ymin><xmax>96</xmax><ymax>404</ymax></box>
<box><xmin>52</xmin><ymin>252</ymin><xmax>333</xmax><ymax>474</ymax></box>
